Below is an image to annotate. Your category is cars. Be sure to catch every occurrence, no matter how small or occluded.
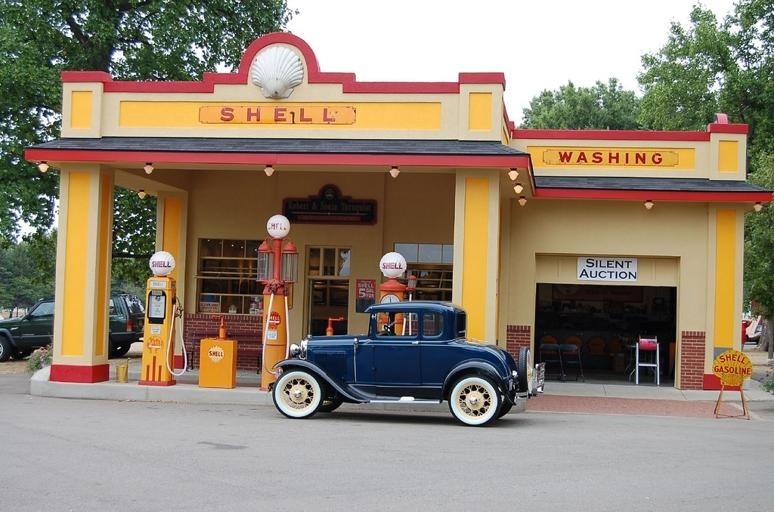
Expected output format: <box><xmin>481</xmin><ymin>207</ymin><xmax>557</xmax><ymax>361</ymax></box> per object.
<box><xmin>742</xmin><ymin>314</ymin><xmax>763</xmax><ymax>342</ymax></box>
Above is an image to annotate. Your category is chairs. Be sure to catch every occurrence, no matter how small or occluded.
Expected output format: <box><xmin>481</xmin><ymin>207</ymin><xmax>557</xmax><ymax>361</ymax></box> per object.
<box><xmin>534</xmin><ymin>329</ymin><xmax>665</xmax><ymax>385</ymax></box>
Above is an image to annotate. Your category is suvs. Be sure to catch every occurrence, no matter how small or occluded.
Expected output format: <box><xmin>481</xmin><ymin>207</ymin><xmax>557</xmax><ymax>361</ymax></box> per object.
<box><xmin>270</xmin><ymin>302</ymin><xmax>547</xmax><ymax>429</ymax></box>
<box><xmin>0</xmin><ymin>294</ymin><xmax>144</xmax><ymax>361</ymax></box>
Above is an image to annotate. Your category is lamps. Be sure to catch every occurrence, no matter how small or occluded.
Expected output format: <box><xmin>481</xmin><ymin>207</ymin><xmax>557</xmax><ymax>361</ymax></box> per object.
<box><xmin>143</xmin><ymin>162</ymin><xmax>154</xmax><ymax>175</ymax></box>
<box><xmin>505</xmin><ymin>167</ymin><xmax>528</xmax><ymax>207</ymax></box>
<box><xmin>137</xmin><ymin>189</ymin><xmax>146</xmax><ymax>200</ymax></box>
<box><xmin>389</xmin><ymin>165</ymin><xmax>400</xmax><ymax>179</ymax></box>
<box><xmin>753</xmin><ymin>202</ymin><xmax>763</xmax><ymax>212</ymax></box>
<box><xmin>38</xmin><ymin>161</ymin><xmax>49</xmax><ymax>173</ymax></box>
<box><xmin>263</xmin><ymin>164</ymin><xmax>275</xmax><ymax>178</ymax></box>
<box><xmin>643</xmin><ymin>200</ymin><xmax>654</xmax><ymax>209</ymax></box>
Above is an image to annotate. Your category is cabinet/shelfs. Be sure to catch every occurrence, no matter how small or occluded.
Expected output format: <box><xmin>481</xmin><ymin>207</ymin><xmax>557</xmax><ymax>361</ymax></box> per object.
<box><xmin>398</xmin><ymin>269</ymin><xmax>453</xmax><ymax>292</ymax></box>
